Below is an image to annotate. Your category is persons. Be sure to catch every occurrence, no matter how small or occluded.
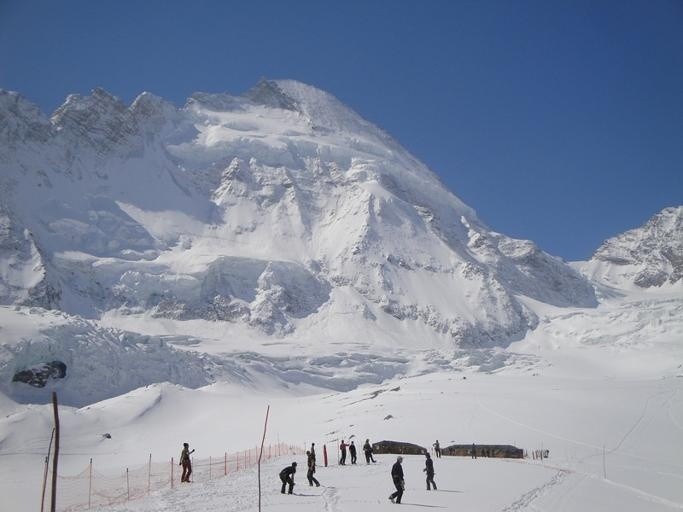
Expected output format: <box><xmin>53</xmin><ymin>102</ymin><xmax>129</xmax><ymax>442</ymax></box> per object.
<box><xmin>179</xmin><ymin>442</ymin><xmax>195</xmax><ymax>483</ymax></box>
<box><xmin>362</xmin><ymin>438</ymin><xmax>376</xmax><ymax>464</ymax></box>
<box><xmin>337</xmin><ymin>439</ymin><xmax>349</xmax><ymax>464</ymax></box>
<box><xmin>310</xmin><ymin>442</ymin><xmax>317</xmax><ymax>466</ymax></box>
<box><xmin>278</xmin><ymin>461</ymin><xmax>297</xmax><ymax>494</ymax></box>
<box><xmin>348</xmin><ymin>440</ymin><xmax>356</xmax><ymax>464</ymax></box>
<box><xmin>470</xmin><ymin>443</ymin><xmax>476</xmax><ymax>459</ymax></box>
<box><xmin>432</xmin><ymin>439</ymin><xmax>441</xmax><ymax>457</ymax></box>
<box><xmin>422</xmin><ymin>452</ymin><xmax>437</xmax><ymax>490</ymax></box>
<box><xmin>305</xmin><ymin>450</ymin><xmax>320</xmax><ymax>487</ymax></box>
<box><xmin>387</xmin><ymin>455</ymin><xmax>405</xmax><ymax>504</ymax></box>
